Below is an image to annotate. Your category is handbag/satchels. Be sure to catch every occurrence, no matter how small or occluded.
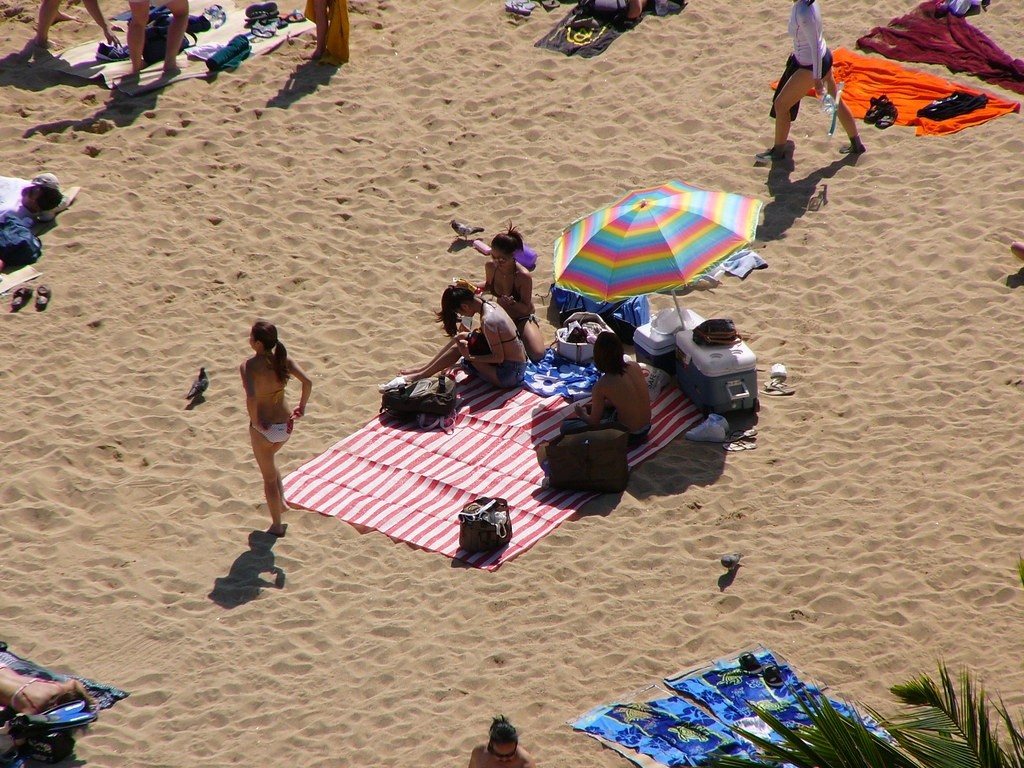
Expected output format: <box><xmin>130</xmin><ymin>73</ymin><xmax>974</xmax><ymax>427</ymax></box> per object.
<box><xmin>458</xmin><ymin>497</ymin><xmax>514</xmax><ymax>551</ymax></box>
<box><xmin>0</xmin><ymin>216</ymin><xmax>41</xmax><ymax>265</ymax></box>
<box><xmin>560</xmin><ymin>295</ymin><xmax>587</xmax><ymax>327</ymax></box>
<box><xmin>538</xmin><ymin>428</ymin><xmax>627</xmax><ymax>492</ymax></box>
<box><xmin>692</xmin><ymin>319</ymin><xmax>743</xmax><ymax>346</ymax></box>
<box><xmin>143</xmin><ymin>15</ymin><xmax>198</xmax><ymax>63</ymax></box>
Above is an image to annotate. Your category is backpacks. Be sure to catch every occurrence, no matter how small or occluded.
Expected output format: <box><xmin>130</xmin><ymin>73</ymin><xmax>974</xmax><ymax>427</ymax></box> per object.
<box><xmin>379</xmin><ymin>375</ymin><xmax>456</xmax><ymax>417</ymax></box>
<box><xmin>621</xmin><ymin>295</ymin><xmax>650</xmax><ymax>326</ymax></box>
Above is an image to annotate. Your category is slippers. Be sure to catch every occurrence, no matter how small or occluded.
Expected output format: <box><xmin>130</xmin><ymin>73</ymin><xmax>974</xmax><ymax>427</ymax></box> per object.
<box><xmin>276</xmin><ymin>12</ymin><xmax>305</xmax><ymax>22</ymax></box>
<box><xmin>722</xmin><ymin>439</ymin><xmax>757</xmax><ymax>451</ymax></box>
<box><xmin>726</xmin><ymin>429</ymin><xmax>757</xmax><ymax>443</ymax></box>
<box><xmin>764</xmin><ymin>379</ymin><xmax>789</xmax><ymax>390</ymax></box>
<box><xmin>763</xmin><ymin>664</ymin><xmax>784</xmax><ymax>687</ymax></box>
<box><xmin>35</xmin><ymin>285</ymin><xmax>50</xmax><ymax>309</ymax></box>
<box><xmin>10</xmin><ymin>288</ymin><xmax>30</xmax><ymax>309</ymax></box>
<box><xmin>739</xmin><ymin>652</ymin><xmax>763</xmax><ymax>675</ymax></box>
<box><xmin>245</xmin><ymin>11</ymin><xmax>279</xmax><ymax>21</ymax></box>
<box><xmin>760</xmin><ymin>387</ymin><xmax>796</xmax><ymax>395</ymax></box>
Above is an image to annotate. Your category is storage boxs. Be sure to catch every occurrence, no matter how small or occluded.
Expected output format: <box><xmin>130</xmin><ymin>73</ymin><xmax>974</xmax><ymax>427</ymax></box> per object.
<box><xmin>555</xmin><ymin>328</ymin><xmax>593</xmax><ymax>362</ymax></box>
<box><xmin>610</xmin><ymin>295</ymin><xmax>677</xmax><ymax>345</ymax></box>
<box><xmin>673</xmin><ymin>330</ymin><xmax>760</xmax><ymax>420</ymax></box>
<box><xmin>632</xmin><ymin>309</ymin><xmax>707</xmax><ymax>373</ymax></box>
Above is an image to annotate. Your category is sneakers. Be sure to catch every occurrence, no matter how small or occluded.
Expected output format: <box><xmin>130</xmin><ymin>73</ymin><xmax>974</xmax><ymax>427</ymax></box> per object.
<box><xmin>686</xmin><ymin>420</ymin><xmax>726</xmax><ymax>442</ymax></box>
<box><xmin>96</xmin><ymin>42</ymin><xmax>129</xmax><ymax>61</ymax></box>
<box><xmin>690</xmin><ymin>413</ymin><xmax>729</xmax><ymax>432</ymax></box>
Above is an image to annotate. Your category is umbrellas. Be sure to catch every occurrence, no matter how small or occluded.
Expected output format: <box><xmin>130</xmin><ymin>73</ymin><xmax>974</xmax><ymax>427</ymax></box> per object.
<box><xmin>550</xmin><ymin>179</ymin><xmax>769</xmax><ymax>332</ymax></box>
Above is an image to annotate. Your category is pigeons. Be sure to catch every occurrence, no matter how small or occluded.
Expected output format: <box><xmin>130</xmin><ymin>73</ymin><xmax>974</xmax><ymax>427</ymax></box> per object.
<box><xmin>185</xmin><ymin>368</ymin><xmax>209</xmax><ymax>400</ymax></box>
<box><xmin>721</xmin><ymin>553</ymin><xmax>744</xmax><ymax>572</ymax></box>
<box><xmin>449</xmin><ymin>219</ymin><xmax>485</xmax><ymax>240</ymax></box>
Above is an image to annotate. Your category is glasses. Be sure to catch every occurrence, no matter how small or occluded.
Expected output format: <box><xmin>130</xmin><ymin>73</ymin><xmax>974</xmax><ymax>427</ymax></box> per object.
<box><xmin>491</xmin><ymin>254</ymin><xmax>509</xmax><ymax>264</ymax></box>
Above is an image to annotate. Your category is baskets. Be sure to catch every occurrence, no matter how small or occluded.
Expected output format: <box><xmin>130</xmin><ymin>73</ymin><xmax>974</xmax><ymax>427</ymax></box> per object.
<box><xmin>612</xmin><ymin>304</ymin><xmax>636</xmax><ymax>344</ymax></box>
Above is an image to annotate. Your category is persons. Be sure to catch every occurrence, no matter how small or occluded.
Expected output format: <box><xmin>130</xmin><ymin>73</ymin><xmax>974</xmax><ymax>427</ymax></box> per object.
<box><xmin>754</xmin><ymin>0</ymin><xmax>867</xmax><ymax>162</ymax></box>
<box><xmin>119</xmin><ymin>0</ymin><xmax>189</xmax><ymax>83</ymax></box>
<box><xmin>560</xmin><ymin>332</ymin><xmax>651</xmax><ymax>446</ymax></box>
<box><xmin>241</xmin><ymin>318</ymin><xmax>314</xmax><ymax>540</ymax></box>
<box><xmin>0</xmin><ymin>171</ymin><xmax>65</xmax><ymax>221</ymax></box>
<box><xmin>468</xmin><ymin>713</ymin><xmax>537</xmax><ymax>768</ymax></box>
<box><xmin>476</xmin><ymin>217</ymin><xmax>548</xmax><ymax>362</ymax></box>
<box><xmin>300</xmin><ymin>0</ymin><xmax>349</xmax><ymax>65</ymax></box>
<box><xmin>398</xmin><ymin>280</ymin><xmax>527</xmax><ymax>388</ymax></box>
<box><xmin>32</xmin><ymin>0</ymin><xmax>123</xmax><ymax>54</ymax></box>
<box><xmin>0</xmin><ymin>648</ymin><xmax>101</xmax><ymax>733</ymax></box>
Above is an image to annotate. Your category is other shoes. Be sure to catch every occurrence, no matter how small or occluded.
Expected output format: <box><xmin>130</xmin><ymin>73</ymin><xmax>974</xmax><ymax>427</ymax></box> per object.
<box><xmin>246</xmin><ymin>2</ymin><xmax>277</xmax><ymax>16</ymax></box>
<box><xmin>754</xmin><ymin>94</ymin><xmax>898</xmax><ymax>163</ymax></box>
